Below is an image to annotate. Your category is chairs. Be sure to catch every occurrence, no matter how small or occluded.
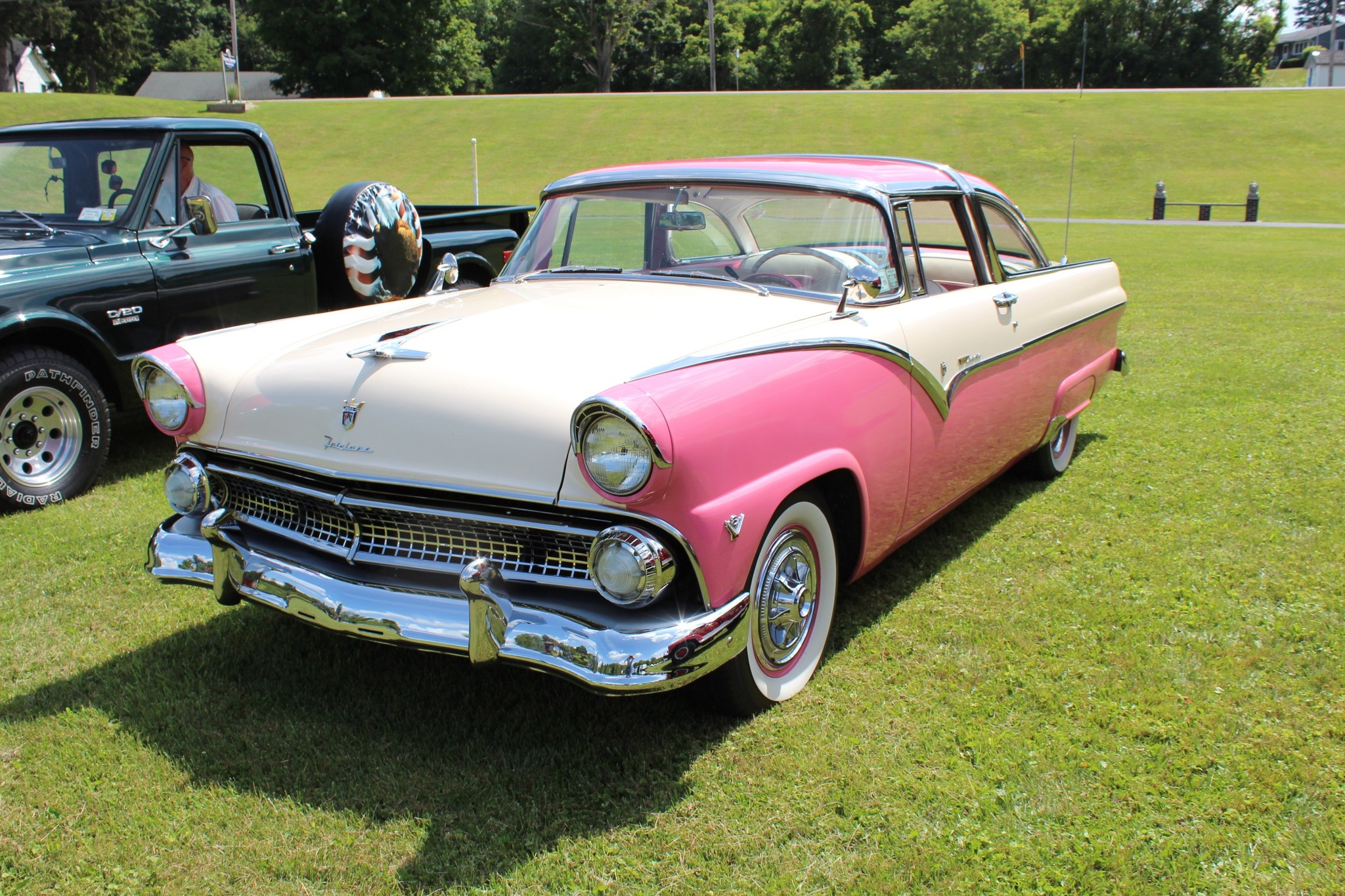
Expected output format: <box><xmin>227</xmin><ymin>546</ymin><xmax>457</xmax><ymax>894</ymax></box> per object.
<box><xmin>234</xmin><ymin>202</ymin><xmax>273</xmax><ymax>222</ymax></box>
<box><xmin>729</xmin><ymin>246</ymin><xmax>1034</xmax><ymax>301</ymax></box>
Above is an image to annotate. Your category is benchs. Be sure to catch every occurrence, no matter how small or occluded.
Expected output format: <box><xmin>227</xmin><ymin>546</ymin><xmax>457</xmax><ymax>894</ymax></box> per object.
<box><xmin>1146</xmin><ymin>179</ymin><xmax>1264</xmax><ymax>224</ymax></box>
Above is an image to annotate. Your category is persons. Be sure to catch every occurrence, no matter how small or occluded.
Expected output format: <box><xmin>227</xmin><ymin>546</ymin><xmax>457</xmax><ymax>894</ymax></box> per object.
<box><xmin>146</xmin><ymin>139</ymin><xmax>240</xmax><ymax>224</ymax></box>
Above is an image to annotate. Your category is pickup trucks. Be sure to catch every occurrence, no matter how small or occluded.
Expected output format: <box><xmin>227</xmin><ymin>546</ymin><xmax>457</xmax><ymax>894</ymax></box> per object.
<box><xmin>132</xmin><ymin>152</ymin><xmax>1132</xmax><ymax>700</ymax></box>
<box><xmin>0</xmin><ymin>116</ymin><xmax>537</xmax><ymax>511</ymax></box>
